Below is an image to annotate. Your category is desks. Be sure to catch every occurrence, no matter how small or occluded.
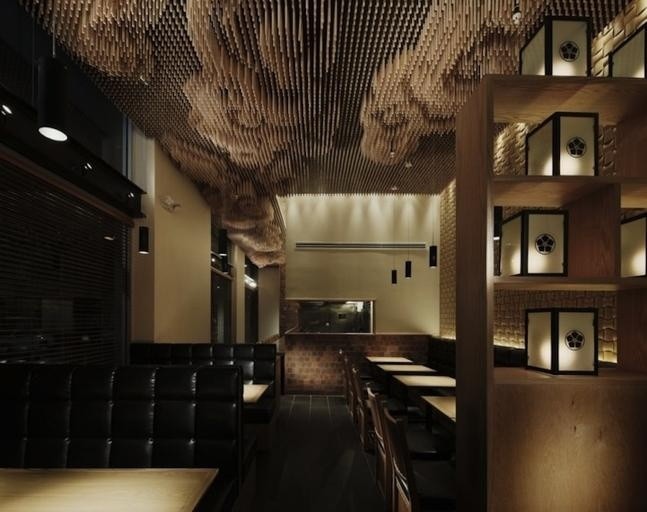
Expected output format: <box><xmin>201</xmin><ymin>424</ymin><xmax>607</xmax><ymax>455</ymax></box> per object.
<box><xmin>242</xmin><ymin>382</ymin><xmax>269</xmax><ymax>404</ymax></box>
<box><xmin>0</xmin><ymin>468</ymin><xmax>221</xmax><ymax>511</ymax></box>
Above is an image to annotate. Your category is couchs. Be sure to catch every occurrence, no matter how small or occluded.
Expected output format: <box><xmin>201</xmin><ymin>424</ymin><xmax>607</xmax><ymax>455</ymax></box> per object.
<box><xmin>242</xmin><ymin>433</ymin><xmax>260</xmax><ymax>482</ymax></box>
<box><xmin>128</xmin><ymin>341</ymin><xmax>274</xmax><ymax>425</ymax></box>
<box><xmin>0</xmin><ymin>358</ymin><xmax>241</xmax><ymax>511</ymax></box>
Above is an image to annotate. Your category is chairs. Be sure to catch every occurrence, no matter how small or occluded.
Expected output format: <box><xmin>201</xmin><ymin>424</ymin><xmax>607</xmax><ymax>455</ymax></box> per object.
<box><xmin>336</xmin><ymin>349</ymin><xmax>458</xmax><ymax>512</ymax></box>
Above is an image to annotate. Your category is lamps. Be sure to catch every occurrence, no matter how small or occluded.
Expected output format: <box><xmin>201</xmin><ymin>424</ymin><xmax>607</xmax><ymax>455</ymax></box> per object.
<box><xmin>429</xmin><ymin>146</ymin><xmax>437</xmax><ymax>266</ymax></box>
<box><xmin>405</xmin><ymin>163</ymin><xmax>411</xmax><ymax>277</ymax></box>
<box><xmin>136</xmin><ymin>226</ymin><xmax>149</xmax><ymax>256</ymax></box>
<box><xmin>391</xmin><ymin>186</ymin><xmax>397</xmax><ymax>283</ymax></box>
<box><xmin>518</xmin><ymin>14</ymin><xmax>593</xmax><ymax>77</ymax></box>
<box><xmin>524</xmin><ymin>307</ymin><xmax>597</xmax><ymax>376</ymax></box>
<box><xmin>498</xmin><ymin>211</ymin><xmax>567</xmax><ymax>278</ymax></box>
<box><xmin>621</xmin><ymin>213</ymin><xmax>647</xmax><ymax>278</ymax></box>
<box><xmin>525</xmin><ymin>112</ymin><xmax>600</xmax><ymax>178</ymax></box>
